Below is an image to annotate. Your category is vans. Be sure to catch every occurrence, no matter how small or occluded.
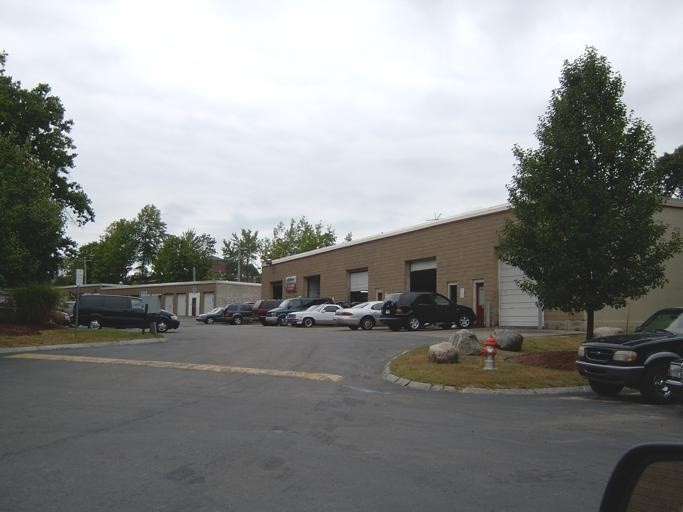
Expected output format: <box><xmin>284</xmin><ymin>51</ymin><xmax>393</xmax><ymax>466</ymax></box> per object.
<box><xmin>69</xmin><ymin>294</ymin><xmax>181</xmax><ymax>334</ymax></box>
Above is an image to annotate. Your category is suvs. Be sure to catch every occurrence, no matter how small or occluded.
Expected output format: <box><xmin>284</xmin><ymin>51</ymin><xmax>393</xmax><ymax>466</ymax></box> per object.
<box><xmin>378</xmin><ymin>291</ymin><xmax>475</xmax><ymax>332</ymax></box>
<box><xmin>252</xmin><ymin>299</ymin><xmax>285</xmax><ymax>325</ymax></box>
<box><xmin>265</xmin><ymin>296</ymin><xmax>334</xmax><ymax>326</ymax></box>
<box><xmin>572</xmin><ymin>306</ymin><xmax>681</xmax><ymax>406</ymax></box>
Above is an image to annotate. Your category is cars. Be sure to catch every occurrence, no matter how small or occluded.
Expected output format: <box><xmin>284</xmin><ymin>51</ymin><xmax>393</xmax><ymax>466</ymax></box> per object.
<box><xmin>333</xmin><ymin>300</ymin><xmax>386</xmax><ymax>330</ymax></box>
<box><xmin>286</xmin><ymin>304</ymin><xmax>346</xmax><ymax>329</ymax></box>
<box><xmin>661</xmin><ymin>356</ymin><xmax>682</xmax><ymax>398</ymax></box>
<box><xmin>195</xmin><ymin>301</ymin><xmax>260</xmax><ymax>325</ymax></box>
<box><xmin>50</xmin><ymin>300</ymin><xmax>75</xmax><ymax>325</ymax></box>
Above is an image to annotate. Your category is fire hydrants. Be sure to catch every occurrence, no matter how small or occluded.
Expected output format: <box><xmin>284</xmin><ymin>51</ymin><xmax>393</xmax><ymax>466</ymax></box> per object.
<box><xmin>479</xmin><ymin>334</ymin><xmax>499</xmax><ymax>371</ymax></box>
